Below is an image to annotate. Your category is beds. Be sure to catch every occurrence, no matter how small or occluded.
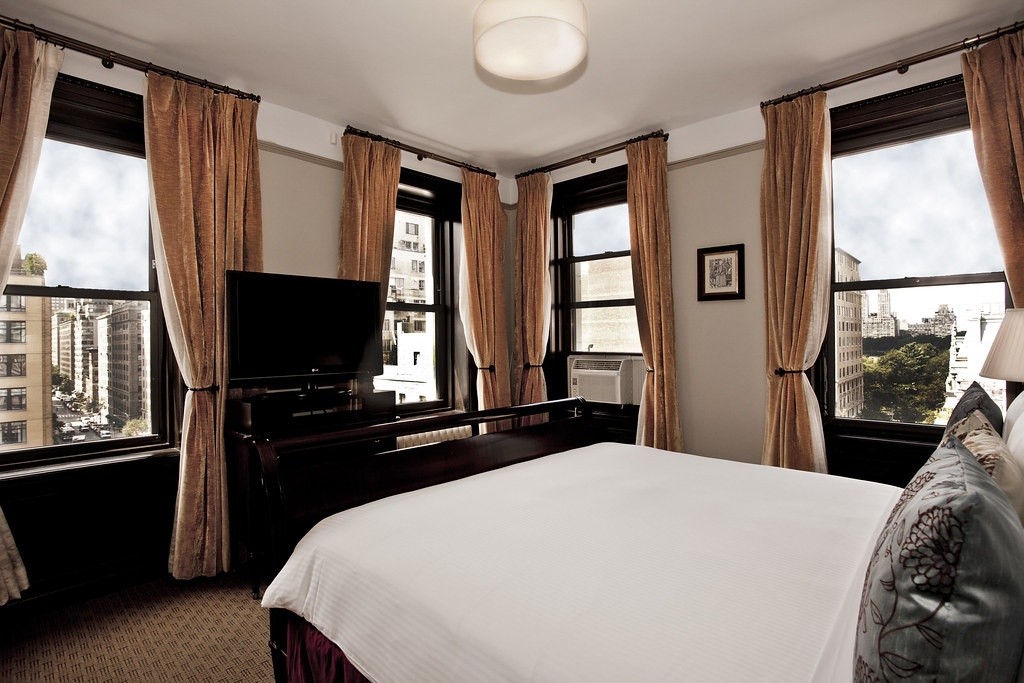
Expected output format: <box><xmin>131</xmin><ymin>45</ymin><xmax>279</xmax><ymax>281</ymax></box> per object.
<box><xmin>261</xmin><ymin>442</ymin><xmax>1024</xmax><ymax>683</ymax></box>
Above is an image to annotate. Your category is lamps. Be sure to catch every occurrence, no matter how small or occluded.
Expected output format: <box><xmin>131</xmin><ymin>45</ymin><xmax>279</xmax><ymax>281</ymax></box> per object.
<box><xmin>979</xmin><ymin>308</ymin><xmax>1024</xmax><ymax>384</ymax></box>
<box><xmin>472</xmin><ymin>0</ymin><xmax>588</xmax><ymax>95</ymax></box>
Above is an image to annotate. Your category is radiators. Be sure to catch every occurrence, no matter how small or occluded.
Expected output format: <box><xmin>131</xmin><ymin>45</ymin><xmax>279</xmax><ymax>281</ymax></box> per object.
<box><xmin>567</xmin><ymin>355</ymin><xmax>646</xmax><ymax>405</ymax></box>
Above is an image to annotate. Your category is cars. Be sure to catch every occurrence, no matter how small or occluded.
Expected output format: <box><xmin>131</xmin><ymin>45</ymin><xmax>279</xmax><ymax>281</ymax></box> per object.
<box><xmin>50</xmin><ymin>389</ymin><xmax>112</xmax><ymax>442</ymax></box>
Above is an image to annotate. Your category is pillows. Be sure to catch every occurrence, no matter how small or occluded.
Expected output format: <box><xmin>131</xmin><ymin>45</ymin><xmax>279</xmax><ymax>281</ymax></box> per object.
<box><xmin>937</xmin><ymin>410</ymin><xmax>1024</xmax><ymax>525</ymax></box>
<box><xmin>853</xmin><ymin>435</ymin><xmax>1024</xmax><ymax>683</ymax></box>
<box><xmin>944</xmin><ymin>381</ymin><xmax>1003</xmax><ymax>437</ymax></box>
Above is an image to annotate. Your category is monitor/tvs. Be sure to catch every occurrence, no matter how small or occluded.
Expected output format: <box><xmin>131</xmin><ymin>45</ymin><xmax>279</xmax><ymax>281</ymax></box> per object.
<box><xmin>225</xmin><ymin>270</ymin><xmax>383</xmax><ymax>403</ymax></box>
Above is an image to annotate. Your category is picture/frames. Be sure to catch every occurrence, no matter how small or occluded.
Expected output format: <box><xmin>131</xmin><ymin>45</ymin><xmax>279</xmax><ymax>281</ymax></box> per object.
<box><xmin>696</xmin><ymin>243</ymin><xmax>746</xmax><ymax>302</ymax></box>
